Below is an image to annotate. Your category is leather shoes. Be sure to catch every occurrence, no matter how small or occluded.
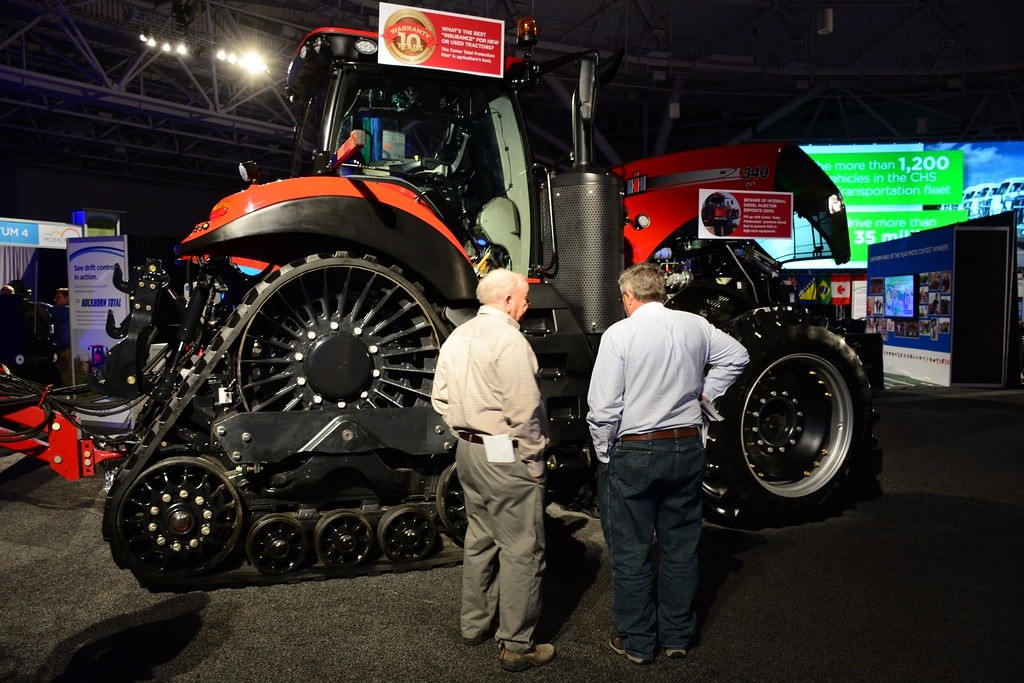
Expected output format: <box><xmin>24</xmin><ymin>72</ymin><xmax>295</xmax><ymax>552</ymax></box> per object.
<box><xmin>618</xmin><ymin>425</ymin><xmax>702</xmax><ymax>441</ymax></box>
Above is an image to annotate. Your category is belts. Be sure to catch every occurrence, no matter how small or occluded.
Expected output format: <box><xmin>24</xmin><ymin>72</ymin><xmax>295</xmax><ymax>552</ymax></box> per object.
<box><xmin>458</xmin><ymin>432</ymin><xmax>518</xmax><ymax>449</ymax></box>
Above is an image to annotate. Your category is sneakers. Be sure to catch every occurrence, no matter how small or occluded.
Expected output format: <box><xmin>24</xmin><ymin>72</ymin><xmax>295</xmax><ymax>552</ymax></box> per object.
<box><xmin>500</xmin><ymin>643</ymin><xmax>555</xmax><ymax>672</ymax></box>
<box><xmin>609</xmin><ymin>632</ymin><xmax>653</xmax><ymax>665</ymax></box>
<box><xmin>665</xmin><ymin>648</ymin><xmax>688</xmax><ymax>658</ymax></box>
<box><xmin>461</xmin><ymin>619</ymin><xmax>499</xmax><ymax>645</ymax></box>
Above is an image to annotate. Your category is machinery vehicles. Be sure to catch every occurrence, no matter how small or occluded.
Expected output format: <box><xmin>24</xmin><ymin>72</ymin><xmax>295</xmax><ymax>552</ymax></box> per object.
<box><xmin>1</xmin><ymin>14</ymin><xmax>880</xmax><ymax>592</ymax></box>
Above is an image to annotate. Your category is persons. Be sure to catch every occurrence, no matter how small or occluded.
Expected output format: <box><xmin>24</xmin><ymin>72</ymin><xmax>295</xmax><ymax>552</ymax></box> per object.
<box><xmin>585</xmin><ymin>262</ymin><xmax>752</xmax><ymax>667</ymax></box>
<box><xmin>429</xmin><ymin>266</ymin><xmax>556</xmax><ymax>672</ymax></box>
<box><xmin>0</xmin><ymin>285</ymin><xmax>72</xmax><ymax>387</ymax></box>
<box><xmin>865</xmin><ymin>271</ymin><xmax>950</xmax><ymax>340</ymax></box>
